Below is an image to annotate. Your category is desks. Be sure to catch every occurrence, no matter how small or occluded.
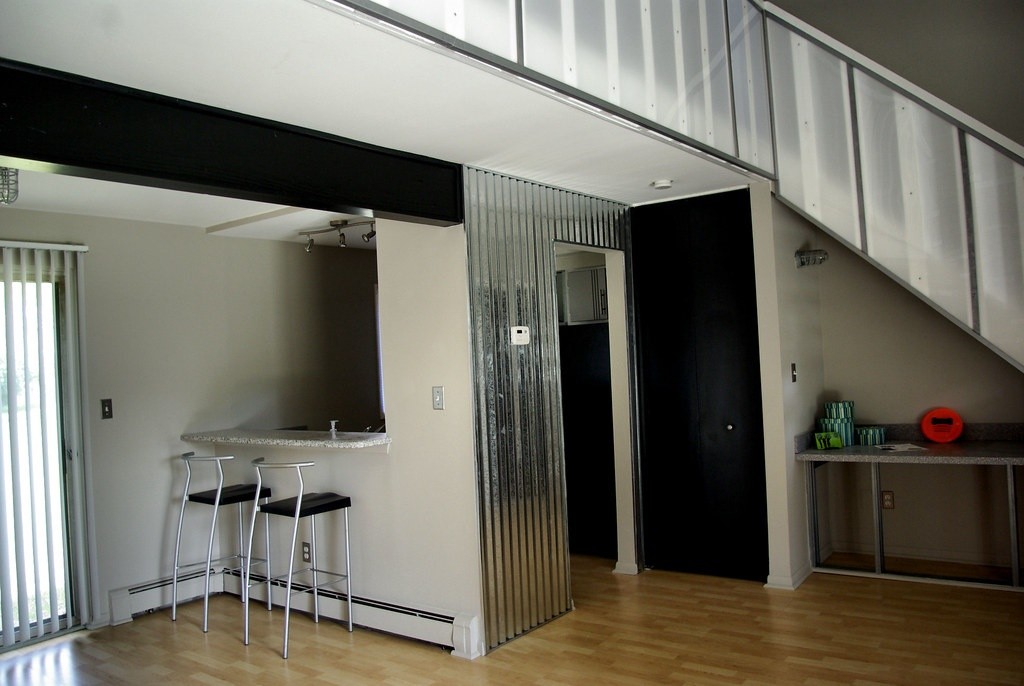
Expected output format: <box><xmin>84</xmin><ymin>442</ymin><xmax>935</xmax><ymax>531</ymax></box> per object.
<box><xmin>799</xmin><ymin>441</ymin><xmax>1022</xmax><ymax>593</ymax></box>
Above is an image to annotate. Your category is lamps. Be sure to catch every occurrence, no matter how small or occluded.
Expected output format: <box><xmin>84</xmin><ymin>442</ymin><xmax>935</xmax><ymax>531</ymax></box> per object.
<box><xmin>0</xmin><ymin>166</ymin><xmax>19</xmax><ymax>206</ymax></box>
<box><xmin>299</xmin><ymin>219</ymin><xmax>376</xmax><ymax>253</ymax></box>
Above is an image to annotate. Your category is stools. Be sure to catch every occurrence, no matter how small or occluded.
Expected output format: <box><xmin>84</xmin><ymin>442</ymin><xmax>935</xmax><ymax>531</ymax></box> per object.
<box><xmin>172</xmin><ymin>452</ymin><xmax>272</xmax><ymax>632</ymax></box>
<box><xmin>244</xmin><ymin>457</ymin><xmax>353</xmax><ymax>660</ymax></box>
